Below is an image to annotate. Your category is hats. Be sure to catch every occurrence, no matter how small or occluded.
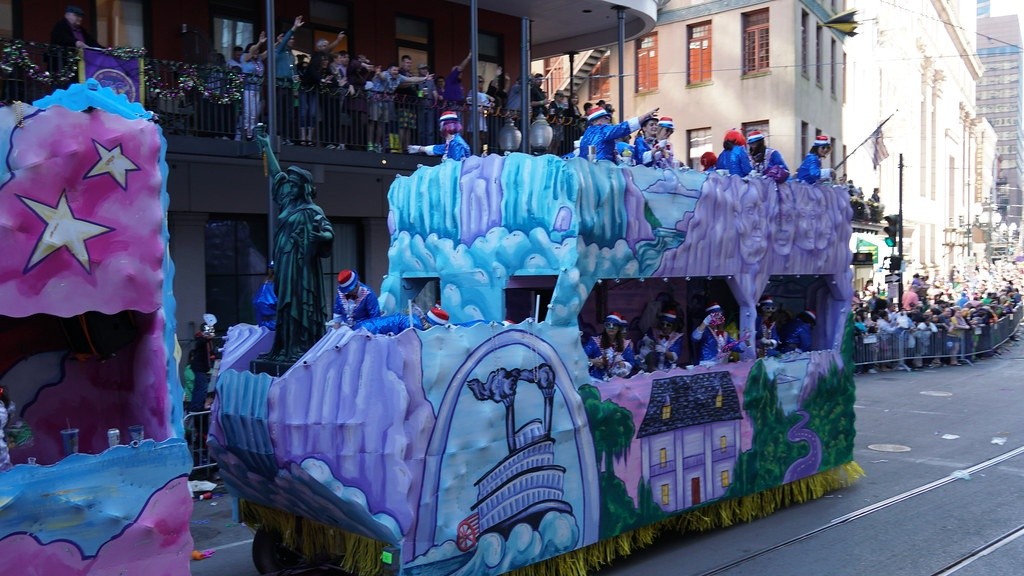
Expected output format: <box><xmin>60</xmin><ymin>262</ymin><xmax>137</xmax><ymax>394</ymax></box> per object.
<box><xmin>641</xmin><ymin>114</ymin><xmax>658</xmax><ymax>123</ymax></box>
<box><xmin>657</xmin><ymin>117</ymin><xmax>673</xmax><ymax>129</ymax></box>
<box><xmin>586</xmin><ymin>107</ymin><xmax>610</xmax><ymax>123</ymax></box>
<box><xmin>596</xmin><ymin>100</ymin><xmax>604</xmax><ymax>105</ymax></box>
<box><xmin>337</xmin><ymin>270</ymin><xmax>358</xmax><ymax>293</ymax></box>
<box><xmin>425</xmin><ymin>308</ymin><xmax>449</xmax><ymax>326</ymax></box>
<box><xmin>705</xmin><ymin>302</ymin><xmax>723</xmax><ymax>317</ymax></box>
<box><xmin>65</xmin><ymin>5</ymin><xmax>86</xmax><ymax>17</ymax></box>
<box><xmin>803</xmin><ymin>307</ymin><xmax>816</xmax><ymax>325</ymax></box>
<box><xmin>604</xmin><ymin>312</ymin><xmax>627</xmax><ymax>326</ymax></box>
<box><xmin>747</xmin><ymin>131</ymin><xmax>765</xmax><ymax>144</ymax></box>
<box><xmin>417</xmin><ymin>64</ymin><xmax>430</xmax><ymax>70</ymax></box>
<box><xmin>439</xmin><ymin>111</ymin><xmax>461</xmax><ymax>123</ymax></box>
<box><xmin>658</xmin><ymin>310</ymin><xmax>676</xmax><ymax>323</ymax></box>
<box><xmin>813</xmin><ymin>135</ymin><xmax>831</xmax><ymax>147</ymax></box>
<box><xmin>758</xmin><ymin>295</ymin><xmax>774</xmax><ymax>306</ymax></box>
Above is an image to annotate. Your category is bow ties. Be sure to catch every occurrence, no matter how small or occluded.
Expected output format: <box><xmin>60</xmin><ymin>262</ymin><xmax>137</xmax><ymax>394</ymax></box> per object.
<box><xmin>606</xmin><ymin>342</ymin><xmax>616</xmax><ymax>350</ymax></box>
<box><xmin>345</xmin><ymin>294</ymin><xmax>358</xmax><ymax>300</ymax></box>
<box><xmin>763</xmin><ymin>320</ymin><xmax>772</xmax><ymax>328</ymax></box>
<box><xmin>716</xmin><ymin>330</ymin><xmax>724</xmax><ymax>337</ymax></box>
<box><xmin>660</xmin><ymin>335</ymin><xmax>670</xmax><ymax>341</ymax></box>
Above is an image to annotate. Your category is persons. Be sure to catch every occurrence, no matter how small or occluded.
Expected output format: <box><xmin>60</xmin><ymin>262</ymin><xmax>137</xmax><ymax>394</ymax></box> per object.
<box><xmin>252</xmin><ymin>259</ymin><xmax>280</xmax><ymax>331</ymax></box>
<box><xmin>199</xmin><ymin>16</ymin><xmax>615</xmax><ymax>158</ymax></box>
<box><xmin>47</xmin><ymin>6</ymin><xmax>112</xmax><ymax>97</ymax></box>
<box><xmin>258</xmin><ymin>132</ymin><xmax>335</xmax><ymax>363</ymax></box>
<box><xmin>325</xmin><ymin>268</ymin><xmax>381</xmax><ymax>333</ymax></box>
<box><xmin>182</xmin><ymin>322</ymin><xmax>238</xmax><ymax>473</ymax></box>
<box><xmin>406</xmin><ymin>111</ymin><xmax>471</xmax><ymax>163</ymax></box>
<box><xmin>352</xmin><ymin>301</ymin><xmax>517</xmax><ymax>337</ymax></box>
<box><xmin>690</xmin><ymin>295</ymin><xmax>816</xmax><ymax>366</ymax></box>
<box><xmin>840</xmin><ymin>174</ymin><xmax>1024</xmax><ymax>376</ymax></box>
<box><xmin>578</xmin><ymin>307</ymin><xmax>686</xmax><ymax>383</ymax></box>
<box><xmin>562</xmin><ymin>105</ymin><xmax>837</xmax><ymax>186</ymax></box>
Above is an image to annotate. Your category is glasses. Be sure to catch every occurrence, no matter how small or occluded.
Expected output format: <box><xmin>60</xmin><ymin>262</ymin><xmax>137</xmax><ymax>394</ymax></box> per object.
<box><xmin>820</xmin><ymin>146</ymin><xmax>830</xmax><ymax>150</ymax></box>
<box><xmin>666</xmin><ymin>129</ymin><xmax>673</xmax><ymax>134</ymax></box>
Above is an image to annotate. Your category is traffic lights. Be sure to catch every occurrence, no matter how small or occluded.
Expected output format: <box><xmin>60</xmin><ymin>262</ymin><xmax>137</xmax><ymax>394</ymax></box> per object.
<box><xmin>882</xmin><ymin>215</ymin><xmax>899</xmax><ymax>247</ymax></box>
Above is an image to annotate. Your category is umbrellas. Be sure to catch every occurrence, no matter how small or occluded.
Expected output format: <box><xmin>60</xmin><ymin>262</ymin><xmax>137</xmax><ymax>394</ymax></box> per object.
<box><xmin>818</xmin><ymin>8</ymin><xmax>878</xmax><ymax>37</ymax></box>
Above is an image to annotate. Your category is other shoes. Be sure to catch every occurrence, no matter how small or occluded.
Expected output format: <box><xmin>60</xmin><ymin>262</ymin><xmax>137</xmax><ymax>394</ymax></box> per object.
<box><xmin>240</xmin><ymin>127</ymin><xmax>403</xmax><ymax>154</ymax></box>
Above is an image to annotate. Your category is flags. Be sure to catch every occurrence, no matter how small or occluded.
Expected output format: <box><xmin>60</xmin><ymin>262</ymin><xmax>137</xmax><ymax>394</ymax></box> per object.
<box><xmin>862</xmin><ymin>127</ymin><xmax>889</xmax><ymax>169</ymax></box>
<box><xmin>78</xmin><ymin>47</ymin><xmax>145</xmax><ymax>109</ymax></box>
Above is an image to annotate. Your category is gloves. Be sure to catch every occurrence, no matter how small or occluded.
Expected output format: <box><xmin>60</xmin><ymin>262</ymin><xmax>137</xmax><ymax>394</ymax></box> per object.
<box><xmin>703</xmin><ymin>315</ymin><xmax>712</xmax><ymax>326</ymax></box>
<box><xmin>643</xmin><ymin>338</ymin><xmax>666</xmax><ymax>354</ymax></box>
<box><xmin>407</xmin><ymin>145</ymin><xmax>421</xmax><ymax>154</ymax></box>
